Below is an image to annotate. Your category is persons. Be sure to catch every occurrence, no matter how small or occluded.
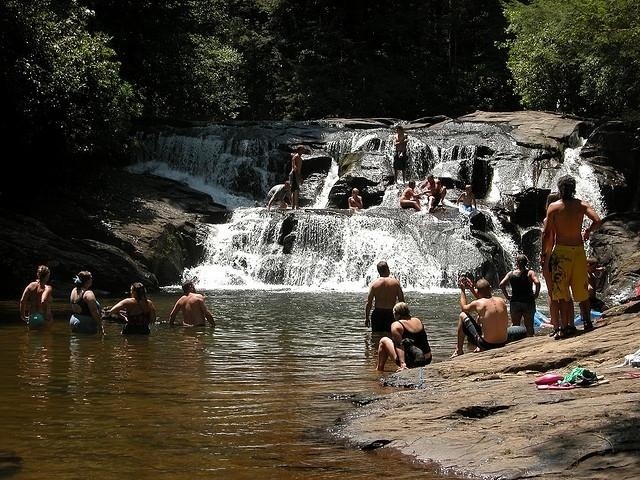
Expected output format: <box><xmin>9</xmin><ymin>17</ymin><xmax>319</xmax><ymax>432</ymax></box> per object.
<box><xmin>457</xmin><ymin>184</ymin><xmax>476</xmax><ymax>212</ymax></box>
<box><xmin>500</xmin><ymin>254</ymin><xmax>540</xmax><ymax>336</ymax></box>
<box><xmin>541</xmin><ymin>191</ymin><xmax>574</xmax><ymax>337</ymax></box>
<box><xmin>449</xmin><ymin>277</ymin><xmax>508</xmax><ymax>358</ymax></box>
<box><xmin>69</xmin><ymin>270</ymin><xmax>105</xmax><ymax>335</ymax></box>
<box><xmin>19</xmin><ymin>265</ymin><xmax>54</xmax><ymax>330</ymax></box>
<box><xmin>587</xmin><ymin>258</ymin><xmax>608</xmax><ymax>313</ymax></box>
<box><xmin>111</xmin><ymin>282</ymin><xmax>156</xmax><ymax>335</ymax></box>
<box><xmin>348</xmin><ymin>187</ymin><xmax>363</xmax><ymax>209</ymax></box>
<box><xmin>364</xmin><ymin>261</ymin><xmax>405</xmax><ymax>331</ymax></box>
<box><xmin>169</xmin><ymin>281</ymin><xmax>217</xmax><ymax>328</ymax></box>
<box><xmin>375</xmin><ymin>302</ymin><xmax>433</xmax><ymax>372</ymax></box>
<box><xmin>289</xmin><ymin>145</ymin><xmax>306</xmax><ymax>210</ymax></box>
<box><xmin>544</xmin><ymin>174</ymin><xmax>601</xmax><ymax>341</ymax></box>
<box><xmin>266</xmin><ymin>181</ymin><xmax>292</xmax><ymax>212</ymax></box>
<box><xmin>418</xmin><ymin>175</ymin><xmax>447</xmax><ymax>212</ymax></box>
<box><xmin>393</xmin><ymin>126</ymin><xmax>409</xmax><ymax>183</ymax></box>
<box><xmin>400</xmin><ymin>179</ymin><xmax>421</xmax><ymax>212</ymax></box>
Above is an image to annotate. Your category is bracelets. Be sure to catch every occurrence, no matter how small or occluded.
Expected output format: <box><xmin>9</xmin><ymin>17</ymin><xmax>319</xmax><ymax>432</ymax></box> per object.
<box><xmin>535</xmin><ymin>293</ymin><xmax>538</xmax><ymax>295</ymax></box>
<box><xmin>400</xmin><ymin>361</ymin><xmax>405</xmax><ymax>364</ymax></box>
<box><xmin>586</xmin><ymin>227</ymin><xmax>592</xmax><ymax>234</ymax></box>
<box><xmin>419</xmin><ymin>193</ymin><xmax>421</xmax><ymax>195</ymax></box>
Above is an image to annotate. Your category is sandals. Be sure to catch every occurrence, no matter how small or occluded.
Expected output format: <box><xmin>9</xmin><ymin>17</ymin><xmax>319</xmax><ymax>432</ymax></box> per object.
<box><xmin>550</xmin><ymin>324</ymin><xmax>593</xmax><ymax>339</ymax></box>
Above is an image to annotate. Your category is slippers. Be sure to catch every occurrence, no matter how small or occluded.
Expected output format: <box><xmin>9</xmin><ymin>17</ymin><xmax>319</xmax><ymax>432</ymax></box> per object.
<box><xmin>538</xmin><ymin>383</ymin><xmax>575</xmax><ymax>390</ymax></box>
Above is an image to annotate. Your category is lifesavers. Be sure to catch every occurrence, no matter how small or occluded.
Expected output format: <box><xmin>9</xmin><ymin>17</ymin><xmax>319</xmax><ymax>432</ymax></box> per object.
<box><xmin>468</xmin><ymin>326</ymin><xmax>527</xmax><ymax>350</ymax></box>
